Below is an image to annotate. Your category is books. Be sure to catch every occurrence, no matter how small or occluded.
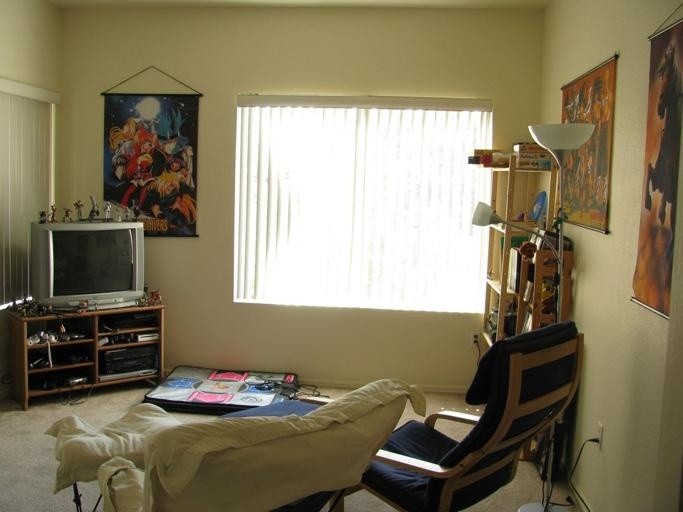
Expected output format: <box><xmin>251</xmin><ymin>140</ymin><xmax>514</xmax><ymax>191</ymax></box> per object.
<box><xmin>505</xmin><ymin>247</ymin><xmax>520</xmax><ymax>293</ymax></box>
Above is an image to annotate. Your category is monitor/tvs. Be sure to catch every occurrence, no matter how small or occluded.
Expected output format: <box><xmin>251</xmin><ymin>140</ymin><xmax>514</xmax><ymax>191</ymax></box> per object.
<box><xmin>30</xmin><ymin>220</ymin><xmax>145</xmax><ymax>311</ymax></box>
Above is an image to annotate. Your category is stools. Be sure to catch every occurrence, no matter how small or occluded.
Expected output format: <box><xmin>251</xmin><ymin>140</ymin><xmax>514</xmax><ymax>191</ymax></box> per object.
<box><xmin>44</xmin><ymin>403</ymin><xmax>181</xmax><ymax>512</ymax></box>
<box><xmin>215</xmin><ymin>401</ymin><xmax>344</xmax><ymax>512</ymax></box>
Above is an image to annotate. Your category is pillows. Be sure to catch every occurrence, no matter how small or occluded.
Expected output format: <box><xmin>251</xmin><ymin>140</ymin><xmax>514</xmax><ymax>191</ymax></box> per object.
<box><xmin>143</xmin><ymin>379</ymin><xmax>426</xmax><ymax>512</ymax></box>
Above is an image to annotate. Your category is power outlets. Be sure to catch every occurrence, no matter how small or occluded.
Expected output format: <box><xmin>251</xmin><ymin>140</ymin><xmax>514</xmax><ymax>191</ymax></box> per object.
<box><xmin>596</xmin><ymin>423</ymin><xmax>603</xmax><ymax>450</ymax></box>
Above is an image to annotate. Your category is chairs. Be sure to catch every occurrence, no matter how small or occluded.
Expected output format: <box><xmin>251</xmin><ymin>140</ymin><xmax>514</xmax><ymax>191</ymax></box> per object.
<box><xmin>345</xmin><ymin>319</ymin><xmax>584</xmax><ymax>512</ymax></box>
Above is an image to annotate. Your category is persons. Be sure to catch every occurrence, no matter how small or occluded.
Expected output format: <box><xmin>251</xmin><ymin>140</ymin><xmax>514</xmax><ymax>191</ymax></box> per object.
<box><xmin>558</xmin><ymin>80</ymin><xmax>612</xmax><ymax>219</ymax></box>
<box><xmin>108</xmin><ymin>115</ymin><xmax>197</xmax><ymax>235</ymax></box>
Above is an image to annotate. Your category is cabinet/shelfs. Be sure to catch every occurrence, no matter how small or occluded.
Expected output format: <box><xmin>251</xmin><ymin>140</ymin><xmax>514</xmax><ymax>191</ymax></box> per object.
<box><xmin>479</xmin><ymin>155</ymin><xmax>558</xmax><ymax>357</ymax></box>
<box><xmin>6</xmin><ymin>304</ymin><xmax>166</xmax><ymax>411</ymax></box>
<box><xmin>514</xmin><ymin>249</ymin><xmax>574</xmax><ymax>461</ymax></box>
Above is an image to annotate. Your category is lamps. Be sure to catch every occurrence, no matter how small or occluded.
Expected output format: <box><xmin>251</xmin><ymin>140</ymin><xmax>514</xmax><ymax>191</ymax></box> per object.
<box><xmin>472</xmin><ymin>122</ymin><xmax>597</xmax><ymax>512</ymax></box>
